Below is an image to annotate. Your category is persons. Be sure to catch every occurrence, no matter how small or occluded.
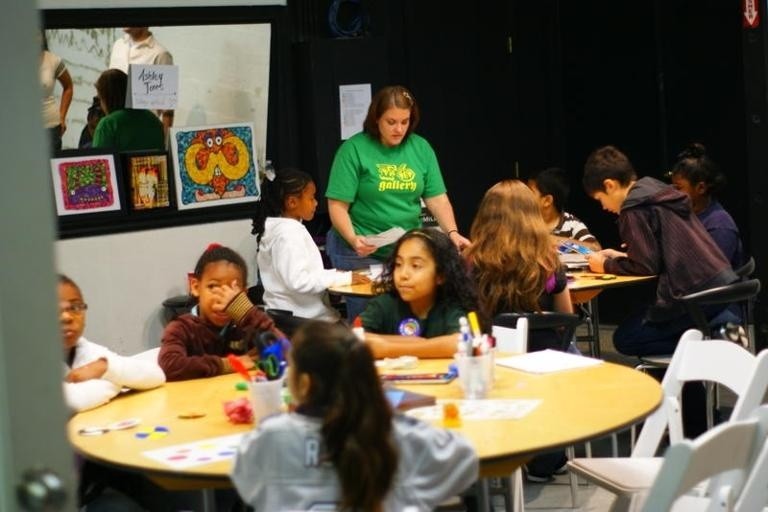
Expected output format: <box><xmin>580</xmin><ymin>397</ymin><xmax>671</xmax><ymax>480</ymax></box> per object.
<box><xmin>251</xmin><ymin>166</ymin><xmax>370</xmax><ymax>334</ymax></box>
<box><xmin>666</xmin><ymin>142</ymin><xmax>744</xmax><ymax>337</ymax></box>
<box><xmin>156</xmin><ymin>244</ymin><xmax>292</xmax><ymax>382</ymax></box>
<box><xmin>37</xmin><ymin>33</ymin><xmax>74</xmax><ymax>160</ymax></box>
<box><xmin>91</xmin><ymin>70</ymin><xmax>167</xmax><ymax>156</ymax></box>
<box><xmin>460</xmin><ymin>182</ymin><xmax>575</xmax><ymax>482</ymax></box>
<box><xmin>232</xmin><ymin>318</ymin><xmax>478</xmax><ymax>511</ymax></box>
<box><xmin>586</xmin><ymin>146</ymin><xmax>749</xmax><ymax>356</ymax></box>
<box><xmin>327</xmin><ymin>86</ymin><xmax>473</xmax><ymax>323</ymax></box>
<box><xmin>53</xmin><ymin>272</ymin><xmax>175</xmax><ymax>512</ymax></box>
<box><xmin>351</xmin><ymin>228</ymin><xmax>476</xmax><ymax>358</ymax></box>
<box><xmin>110</xmin><ymin>27</ymin><xmax>176</xmax><ymax>145</ymax></box>
<box><xmin>525</xmin><ymin>168</ymin><xmax>603</xmax><ymax>252</ymax></box>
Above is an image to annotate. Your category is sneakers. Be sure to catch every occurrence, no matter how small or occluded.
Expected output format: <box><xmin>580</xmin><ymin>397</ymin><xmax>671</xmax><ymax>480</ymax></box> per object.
<box><xmin>524</xmin><ymin>453</ymin><xmax>568</xmax><ymax>483</ymax></box>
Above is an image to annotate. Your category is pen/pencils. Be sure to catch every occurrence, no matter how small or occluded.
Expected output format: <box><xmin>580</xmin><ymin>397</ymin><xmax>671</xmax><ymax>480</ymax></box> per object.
<box><xmin>231</xmin><ymin>355</ymin><xmax>253</xmax><ymax>383</ymax></box>
<box><xmin>455</xmin><ymin>308</ymin><xmax>495</xmax><ymax>359</ymax></box>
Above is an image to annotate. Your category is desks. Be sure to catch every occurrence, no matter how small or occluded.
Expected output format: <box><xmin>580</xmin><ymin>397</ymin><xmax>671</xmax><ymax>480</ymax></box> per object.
<box><xmin>327</xmin><ymin>283</ymin><xmax>391</xmax><ymax>299</ymax></box>
<box><xmin>65</xmin><ymin>350</ymin><xmax>665</xmax><ymax>510</ymax></box>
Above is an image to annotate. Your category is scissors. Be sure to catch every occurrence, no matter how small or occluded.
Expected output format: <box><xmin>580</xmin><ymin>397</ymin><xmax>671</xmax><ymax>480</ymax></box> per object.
<box><xmin>254</xmin><ymin>353</ymin><xmax>281</xmax><ymax>380</ymax></box>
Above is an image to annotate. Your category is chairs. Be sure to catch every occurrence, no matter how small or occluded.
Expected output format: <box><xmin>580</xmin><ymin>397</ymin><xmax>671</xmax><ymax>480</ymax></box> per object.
<box><xmin>487</xmin><ymin>253</ymin><xmax>766</xmax><ymax>510</ymax></box>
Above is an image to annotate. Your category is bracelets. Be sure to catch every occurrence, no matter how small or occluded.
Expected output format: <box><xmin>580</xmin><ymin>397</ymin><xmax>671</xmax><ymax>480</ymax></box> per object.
<box><xmin>446</xmin><ymin>230</ymin><xmax>459</xmax><ymax>236</ymax></box>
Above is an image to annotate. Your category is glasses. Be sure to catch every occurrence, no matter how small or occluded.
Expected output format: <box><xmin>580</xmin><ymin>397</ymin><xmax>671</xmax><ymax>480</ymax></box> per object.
<box><xmin>60</xmin><ymin>302</ymin><xmax>88</xmax><ymax>316</ymax></box>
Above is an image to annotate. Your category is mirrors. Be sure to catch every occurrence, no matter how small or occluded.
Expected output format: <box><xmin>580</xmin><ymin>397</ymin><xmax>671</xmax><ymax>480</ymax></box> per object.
<box><xmin>39</xmin><ymin>3</ymin><xmax>295</xmax><ymax>223</ymax></box>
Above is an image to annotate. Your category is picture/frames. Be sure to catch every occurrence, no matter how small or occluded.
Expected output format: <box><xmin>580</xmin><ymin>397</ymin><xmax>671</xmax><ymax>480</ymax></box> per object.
<box><xmin>168</xmin><ymin>121</ymin><xmax>263</xmax><ymax>211</ymax></box>
<box><xmin>118</xmin><ymin>146</ymin><xmax>172</xmax><ymax>213</ymax></box>
<box><xmin>47</xmin><ymin>149</ymin><xmax>126</xmax><ymax>222</ymax></box>
